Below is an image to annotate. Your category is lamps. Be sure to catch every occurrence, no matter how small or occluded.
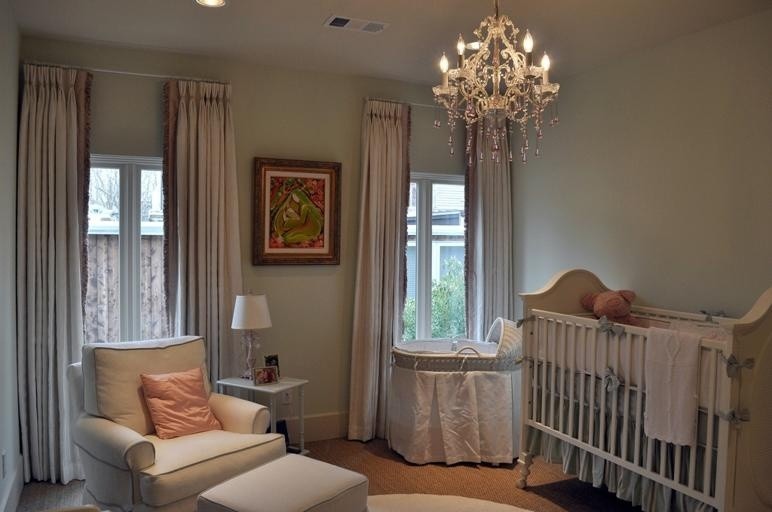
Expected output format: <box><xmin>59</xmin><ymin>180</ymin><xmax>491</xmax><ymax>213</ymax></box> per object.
<box><xmin>231</xmin><ymin>288</ymin><xmax>272</xmax><ymax>379</ymax></box>
<box><xmin>430</xmin><ymin>0</ymin><xmax>561</xmax><ymax>168</ymax></box>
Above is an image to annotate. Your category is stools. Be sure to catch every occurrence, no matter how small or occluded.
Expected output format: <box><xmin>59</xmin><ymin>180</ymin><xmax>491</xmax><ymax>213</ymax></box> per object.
<box><xmin>197</xmin><ymin>452</ymin><xmax>369</xmax><ymax>512</ymax></box>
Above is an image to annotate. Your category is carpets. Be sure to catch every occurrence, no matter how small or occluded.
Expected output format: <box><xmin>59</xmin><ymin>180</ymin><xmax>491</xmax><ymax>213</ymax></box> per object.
<box><xmin>367</xmin><ymin>493</ymin><xmax>534</xmax><ymax>512</ymax></box>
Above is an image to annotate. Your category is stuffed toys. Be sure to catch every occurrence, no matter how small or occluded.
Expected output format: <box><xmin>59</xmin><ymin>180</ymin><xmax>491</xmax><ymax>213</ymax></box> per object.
<box><xmin>580</xmin><ymin>286</ymin><xmax>642</xmax><ymax>327</ymax></box>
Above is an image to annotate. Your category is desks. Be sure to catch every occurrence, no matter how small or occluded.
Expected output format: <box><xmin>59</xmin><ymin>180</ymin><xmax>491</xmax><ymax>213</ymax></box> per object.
<box><xmin>216</xmin><ymin>375</ymin><xmax>311</xmax><ymax>456</ymax></box>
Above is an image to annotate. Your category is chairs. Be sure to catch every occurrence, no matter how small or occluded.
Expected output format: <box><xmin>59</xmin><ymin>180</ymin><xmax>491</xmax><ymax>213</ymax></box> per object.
<box><xmin>64</xmin><ymin>335</ymin><xmax>286</xmax><ymax>511</ymax></box>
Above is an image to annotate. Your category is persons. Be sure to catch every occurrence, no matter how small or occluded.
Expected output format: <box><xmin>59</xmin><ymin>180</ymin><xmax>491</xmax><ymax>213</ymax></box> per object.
<box><xmin>255</xmin><ymin>368</ymin><xmax>277</xmax><ymax>384</ymax></box>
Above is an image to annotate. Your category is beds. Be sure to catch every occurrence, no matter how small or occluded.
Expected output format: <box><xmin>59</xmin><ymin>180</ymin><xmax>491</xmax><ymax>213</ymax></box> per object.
<box><xmin>516</xmin><ymin>267</ymin><xmax>770</xmax><ymax>512</ymax></box>
<box><xmin>391</xmin><ymin>317</ymin><xmax>524</xmax><ymax>466</ymax></box>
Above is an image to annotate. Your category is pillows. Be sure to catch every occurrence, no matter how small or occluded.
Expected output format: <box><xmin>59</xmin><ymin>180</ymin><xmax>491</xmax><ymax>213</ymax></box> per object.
<box><xmin>137</xmin><ymin>367</ymin><xmax>224</xmax><ymax>441</ymax></box>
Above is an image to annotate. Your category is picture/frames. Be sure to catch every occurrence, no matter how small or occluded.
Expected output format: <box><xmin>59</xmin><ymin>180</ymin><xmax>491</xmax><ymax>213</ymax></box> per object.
<box><xmin>252</xmin><ymin>366</ymin><xmax>280</xmax><ymax>387</ymax></box>
<box><xmin>251</xmin><ymin>155</ymin><xmax>342</xmax><ymax>267</ymax></box>
